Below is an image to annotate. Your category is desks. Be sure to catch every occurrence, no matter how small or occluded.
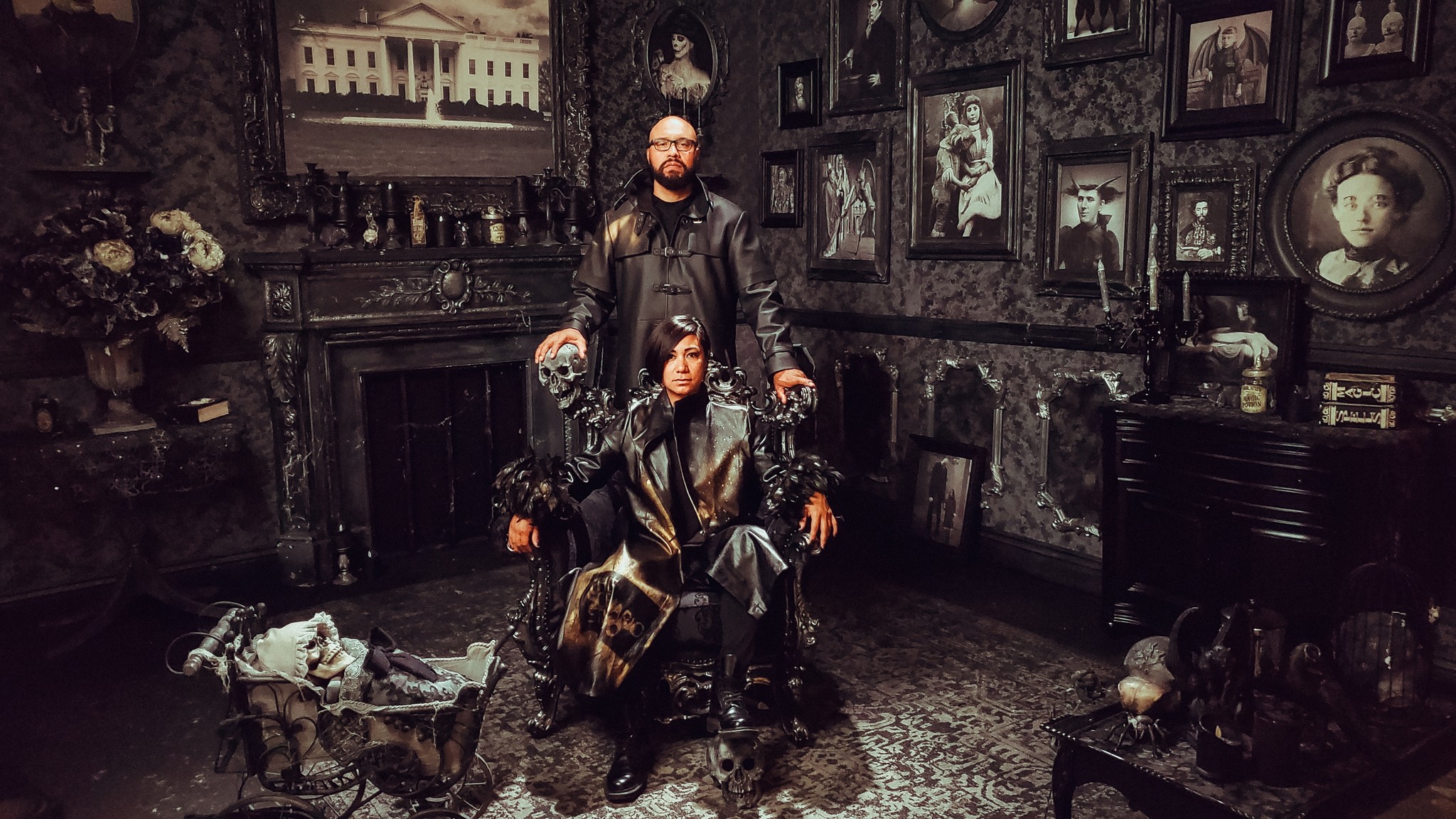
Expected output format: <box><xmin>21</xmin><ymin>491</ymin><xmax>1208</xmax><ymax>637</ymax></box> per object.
<box><xmin>1</xmin><ymin>415</ymin><xmax>257</xmax><ymax>659</ymax></box>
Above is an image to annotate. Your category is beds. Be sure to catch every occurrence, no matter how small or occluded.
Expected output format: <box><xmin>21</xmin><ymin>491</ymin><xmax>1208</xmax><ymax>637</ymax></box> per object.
<box><xmin>165</xmin><ymin>580</ymin><xmax>535</xmax><ymax>819</ymax></box>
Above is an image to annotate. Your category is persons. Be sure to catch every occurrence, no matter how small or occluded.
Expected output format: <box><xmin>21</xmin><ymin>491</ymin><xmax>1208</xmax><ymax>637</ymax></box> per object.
<box><xmin>510</xmin><ymin>315</ymin><xmax>845</xmax><ymax>794</ymax></box>
<box><xmin>533</xmin><ymin>114</ymin><xmax>820</xmax><ymax>403</ymax></box>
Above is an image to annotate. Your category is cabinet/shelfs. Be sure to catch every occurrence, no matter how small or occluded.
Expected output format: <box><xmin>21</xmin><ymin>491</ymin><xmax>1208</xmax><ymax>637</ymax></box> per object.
<box><xmin>1097</xmin><ymin>392</ymin><xmax>1456</xmax><ymax>646</ymax></box>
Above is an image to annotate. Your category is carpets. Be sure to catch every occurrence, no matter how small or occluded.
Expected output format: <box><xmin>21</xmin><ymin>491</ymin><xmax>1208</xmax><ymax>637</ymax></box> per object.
<box><xmin>265</xmin><ymin>577</ymin><xmax>1322</xmax><ymax>819</ymax></box>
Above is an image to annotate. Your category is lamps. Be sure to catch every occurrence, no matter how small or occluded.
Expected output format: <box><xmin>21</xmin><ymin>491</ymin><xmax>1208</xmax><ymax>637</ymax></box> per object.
<box><xmin>35</xmin><ymin>44</ymin><xmax>118</xmax><ymax>167</ymax></box>
<box><xmin>667</xmin><ymin>83</ymin><xmax>703</xmax><ymax>153</ymax></box>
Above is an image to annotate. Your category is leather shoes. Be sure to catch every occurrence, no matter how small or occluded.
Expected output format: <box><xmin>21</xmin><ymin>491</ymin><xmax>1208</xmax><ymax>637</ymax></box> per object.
<box><xmin>604</xmin><ymin>727</ymin><xmax>657</xmax><ymax>803</ymax></box>
<box><xmin>716</xmin><ymin>679</ymin><xmax>760</xmax><ymax>741</ymax></box>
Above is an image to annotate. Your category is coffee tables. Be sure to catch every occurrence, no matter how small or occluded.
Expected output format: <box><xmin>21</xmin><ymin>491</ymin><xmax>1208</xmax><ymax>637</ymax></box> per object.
<box><xmin>1040</xmin><ymin>666</ymin><xmax>1456</xmax><ymax>819</ymax></box>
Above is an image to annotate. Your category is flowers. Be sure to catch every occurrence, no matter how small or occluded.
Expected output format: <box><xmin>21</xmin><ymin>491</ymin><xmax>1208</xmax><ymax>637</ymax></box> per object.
<box><xmin>0</xmin><ymin>199</ymin><xmax>233</xmax><ymax>355</ymax></box>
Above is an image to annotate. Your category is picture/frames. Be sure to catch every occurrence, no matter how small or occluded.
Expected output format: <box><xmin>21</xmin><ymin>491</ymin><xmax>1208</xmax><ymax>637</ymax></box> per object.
<box><xmin>631</xmin><ymin>0</ymin><xmax>731</xmax><ymax>130</ymax></box>
<box><xmin>758</xmin><ymin>0</ymin><xmax>1455</xmax><ymax>573</ymax></box>
<box><xmin>232</xmin><ymin>1</ymin><xmax>593</xmax><ymax>224</ymax></box>
<box><xmin>0</xmin><ymin>0</ymin><xmax>164</xmax><ymax>118</ymax></box>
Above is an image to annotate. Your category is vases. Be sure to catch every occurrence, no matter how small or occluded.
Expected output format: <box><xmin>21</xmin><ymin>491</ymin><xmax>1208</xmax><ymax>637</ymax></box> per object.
<box><xmin>82</xmin><ymin>335</ymin><xmax>160</xmax><ymax>437</ymax></box>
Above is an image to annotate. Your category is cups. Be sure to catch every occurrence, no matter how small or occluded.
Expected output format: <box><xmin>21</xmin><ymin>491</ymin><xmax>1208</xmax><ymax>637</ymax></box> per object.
<box><xmin>32</xmin><ymin>397</ymin><xmax>58</xmax><ymax>435</ymax></box>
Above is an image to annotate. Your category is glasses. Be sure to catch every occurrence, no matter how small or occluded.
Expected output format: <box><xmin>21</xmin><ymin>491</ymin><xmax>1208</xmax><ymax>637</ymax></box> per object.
<box><xmin>647</xmin><ymin>139</ymin><xmax>697</xmax><ymax>151</ymax></box>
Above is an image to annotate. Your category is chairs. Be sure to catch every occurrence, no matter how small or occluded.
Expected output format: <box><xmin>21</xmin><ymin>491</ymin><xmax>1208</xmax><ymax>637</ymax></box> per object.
<box><xmin>483</xmin><ymin>343</ymin><xmax>845</xmax><ymax>749</ymax></box>
